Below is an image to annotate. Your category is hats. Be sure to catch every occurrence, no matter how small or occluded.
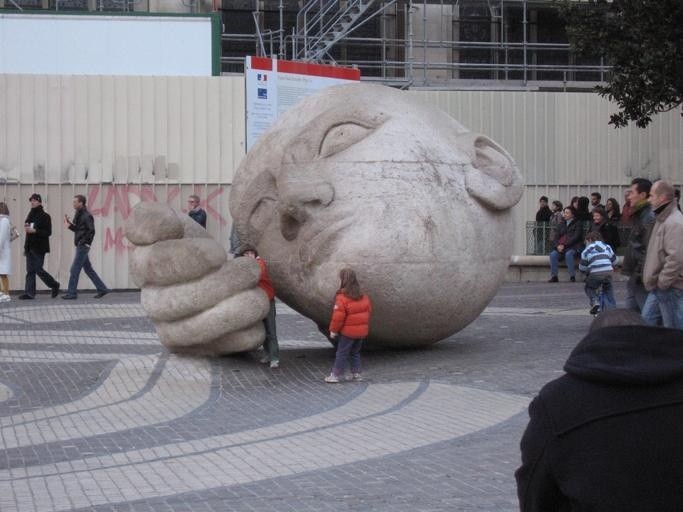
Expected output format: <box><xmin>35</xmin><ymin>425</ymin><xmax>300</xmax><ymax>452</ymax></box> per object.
<box><xmin>239</xmin><ymin>243</ymin><xmax>256</xmax><ymax>256</ymax></box>
<box><xmin>29</xmin><ymin>193</ymin><xmax>42</xmax><ymax>202</ymax></box>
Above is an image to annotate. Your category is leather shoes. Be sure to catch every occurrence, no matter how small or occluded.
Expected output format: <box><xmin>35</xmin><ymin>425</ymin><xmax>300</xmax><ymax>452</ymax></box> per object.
<box><xmin>51</xmin><ymin>281</ymin><xmax>60</xmax><ymax>297</ymax></box>
<box><xmin>61</xmin><ymin>294</ymin><xmax>77</xmax><ymax>300</ymax></box>
<box><xmin>19</xmin><ymin>294</ymin><xmax>34</xmax><ymax>299</ymax></box>
<box><xmin>94</xmin><ymin>289</ymin><xmax>110</xmax><ymax>298</ymax></box>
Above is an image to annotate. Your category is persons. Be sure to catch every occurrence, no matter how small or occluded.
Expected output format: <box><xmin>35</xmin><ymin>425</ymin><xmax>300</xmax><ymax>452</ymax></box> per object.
<box><xmin>229</xmin><ymin>219</ymin><xmax>241</xmax><ymax>258</ymax></box>
<box><xmin>186</xmin><ymin>195</ymin><xmax>206</xmax><ymax>231</ymax></box>
<box><xmin>240</xmin><ymin>243</ymin><xmax>280</xmax><ymax>368</ymax></box>
<box><xmin>324</xmin><ymin>268</ymin><xmax>372</xmax><ymax>383</ymax></box>
<box><xmin>19</xmin><ymin>194</ymin><xmax>60</xmax><ymax>300</ymax></box>
<box><xmin>60</xmin><ymin>195</ymin><xmax>109</xmax><ymax>299</ymax></box>
<box><xmin>0</xmin><ymin>202</ymin><xmax>12</xmax><ymax>303</ymax></box>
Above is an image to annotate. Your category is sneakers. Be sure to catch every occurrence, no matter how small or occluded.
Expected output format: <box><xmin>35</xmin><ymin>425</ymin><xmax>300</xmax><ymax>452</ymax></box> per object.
<box><xmin>0</xmin><ymin>291</ymin><xmax>11</xmax><ymax>303</ymax></box>
<box><xmin>324</xmin><ymin>372</ymin><xmax>341</xmax><ymax>383</ymax></box>
<box><xmin>548</xmin><ymin>276</ymin><xmax>558</xmax><ymax>282</ymax></box>
<box><xmin>589</xmin><ymin>300</ymin><xmax>600</xmax><ymax>315</ymax></box>
<box><xmin>260</xmin><ymin>354</ymin><xmax>270</xmax><ymax>363</ymax></box>
<box><xmin>269</xmin><ymin>359</ymin><xmax>280</xmax><ymax>368</ymax></box>
<box><xmin>569</xmin><ymin>276</ymin><xmax>575</xmax><ymax>282</ymax></box>
<box><xmin>344</xmin><ymin>372</ymin><xmax>362</xmax><ymax>381</ymax></box>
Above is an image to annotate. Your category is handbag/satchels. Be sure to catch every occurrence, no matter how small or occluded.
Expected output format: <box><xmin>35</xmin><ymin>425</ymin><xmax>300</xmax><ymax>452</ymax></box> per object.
<box><xmin>9</xmin><ymin>222</ymin><xmax>20</xmax><ymax>241</ymax></box>
<box><xmin>558</xmin><ymin>234</ymin><xmax>571</xmax><ymax>245</ymax></box>
